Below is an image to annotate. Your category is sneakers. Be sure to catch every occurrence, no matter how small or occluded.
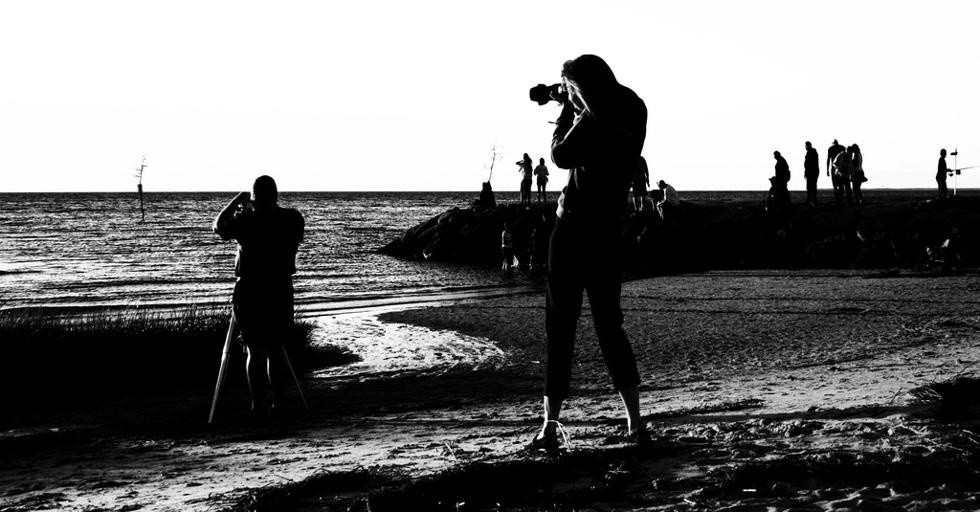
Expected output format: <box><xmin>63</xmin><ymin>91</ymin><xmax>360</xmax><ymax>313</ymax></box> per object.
<box><xmin>524</xmin><ymin>434</ymin><xmax>557</xmax><ymax>448</ymax></box>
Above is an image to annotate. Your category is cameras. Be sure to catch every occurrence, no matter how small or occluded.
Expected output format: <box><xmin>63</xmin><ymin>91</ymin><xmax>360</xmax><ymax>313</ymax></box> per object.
<box><xmin>529</xmin><ymin>83</ymin><xmax>568</xmax><ymax>110</ymax></box>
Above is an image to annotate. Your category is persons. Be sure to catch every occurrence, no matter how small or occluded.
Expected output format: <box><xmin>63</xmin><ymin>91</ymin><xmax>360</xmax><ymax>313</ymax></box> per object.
<box><xmin>656</xmin><ymin>179</ymin><xmax>679</xmax><ymax>221</ymax></box>
<box><xmin>515</xmin><ymin>153</ymin><xmax>534</xmax><ymax>203</ymax></box>
<box><xmin>935</xmin><ymin>148</ymin><xmax>953</xmax><ymax>190</ymax></box>
<box><xmin>627</xmin><ymin>154</ymin><xmax>650</xmax><ymax>217</ymax></box>
<box><xmin>768</xmin><ymin>138</ymin><xmax>868</xmax><ymax>204</ymax></box>
<box><xmin>500</xmin><ymin>221</ymin><xmax>517</xmax><ymax>281</ymax></box>
<box><xmin>212</xmin><ymin>172</ymin><xmax>308</xmax><ymax>435</ymax></box>
<box><xmin>534</xmin><ymin>157</ymin><xmax>550</xmax><ymax>203</ymax></box>
<box><xmin>520</xmin><ymin>51</ymin><xmax>649</xmax><ymax>450</ymax></box>
